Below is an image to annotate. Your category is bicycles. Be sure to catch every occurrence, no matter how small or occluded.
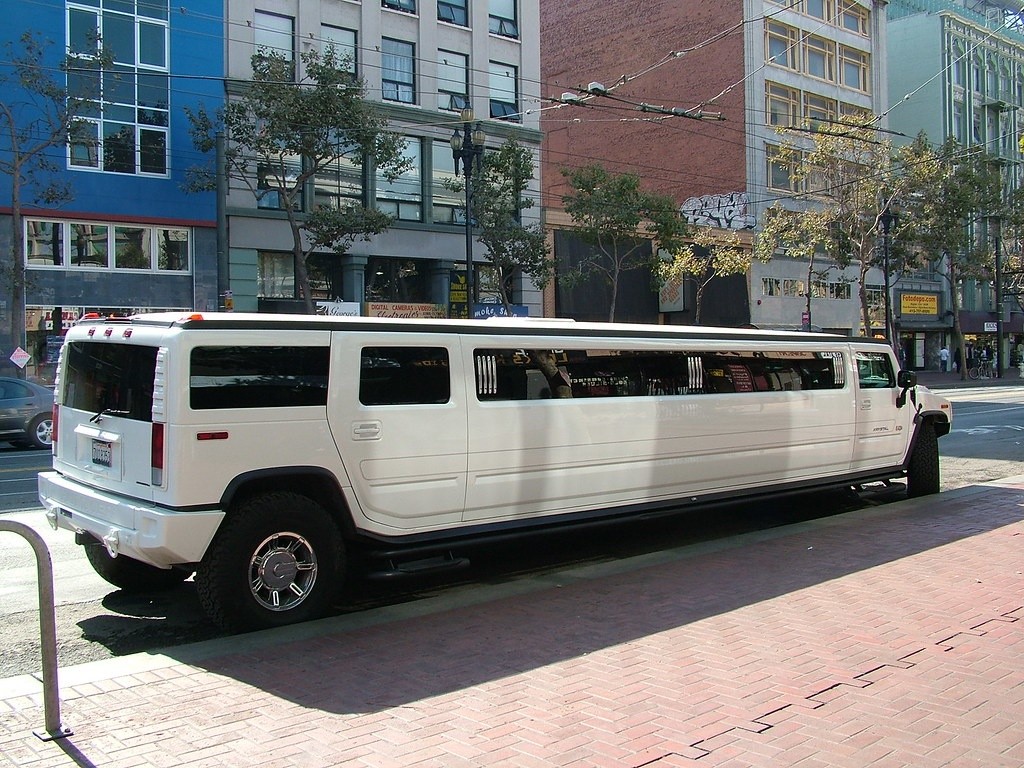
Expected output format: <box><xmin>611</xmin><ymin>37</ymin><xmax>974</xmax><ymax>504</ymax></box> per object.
<box><xmin>969</xmin><ymin>355</ymin><xmax>994</xmax><ymax>380</ymax></box>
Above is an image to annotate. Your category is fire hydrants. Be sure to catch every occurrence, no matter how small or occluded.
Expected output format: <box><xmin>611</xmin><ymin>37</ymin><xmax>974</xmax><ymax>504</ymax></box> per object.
<box><xmin>1018</xmin><ymin>363</ymin><xmax>1024</xmax><ymax>379</ymax></box>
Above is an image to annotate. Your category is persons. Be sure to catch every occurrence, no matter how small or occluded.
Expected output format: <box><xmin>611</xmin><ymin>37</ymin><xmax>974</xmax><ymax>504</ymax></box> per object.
<box><xmin>898</xmin><ymin>342</ymin><xmax>906</xmax><ymax>370</ymax></box>
<box><xmin>939</xmin><ymin>345</ymin><xmax>950</xmax><ymax>374</ymax></box>
<box><xmin>953</xmin><ymin>347</ymin><xmax>962</xmax><ymax>374</ymax></box>
<box><xmin>980</xmin><ymin>344</ymin><xmax>999</xmax><ymax>381</ymax></box>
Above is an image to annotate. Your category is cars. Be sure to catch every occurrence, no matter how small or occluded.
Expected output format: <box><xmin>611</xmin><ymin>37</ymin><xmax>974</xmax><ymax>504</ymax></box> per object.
<box><xmin>0</xmin><ymin>348</ymin><xmax>14</xmax><ymax>368</ymax></box>
<box><xmin>0</xmin><ymin>376</ymin><xmax>54</xmax><ymax>449</ymax></box>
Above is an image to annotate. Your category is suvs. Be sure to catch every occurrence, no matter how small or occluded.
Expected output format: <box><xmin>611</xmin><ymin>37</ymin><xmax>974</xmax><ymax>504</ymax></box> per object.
<box><xmin>38</xmin><ymin>308</ymin><xmax>951</xmax><ymax>633</ymax></box>
<box><xmin>45</xmin><ymin>336</ymin><xmax>65</xmax><ymax>362</ymax></box>
